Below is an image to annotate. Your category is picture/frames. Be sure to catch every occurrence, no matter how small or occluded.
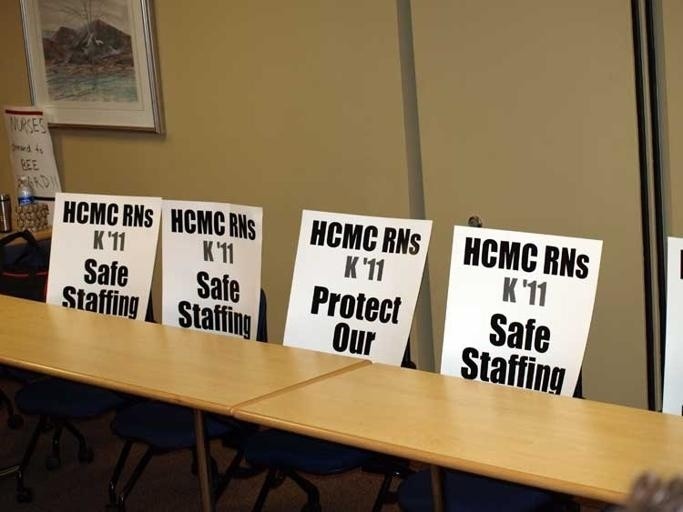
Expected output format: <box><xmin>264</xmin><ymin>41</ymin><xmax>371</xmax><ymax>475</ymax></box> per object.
<box><xmin>20</xmin><ymin>0</ymin><xmax>166</xmax><ymax>135</ymax></box>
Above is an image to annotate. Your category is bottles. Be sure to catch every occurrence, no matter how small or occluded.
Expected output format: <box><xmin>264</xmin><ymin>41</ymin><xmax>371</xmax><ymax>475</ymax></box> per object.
<box><xmin>17</xmin><ymin>176</ymin><xmax>33</xmax><ymax>206</ymax></box>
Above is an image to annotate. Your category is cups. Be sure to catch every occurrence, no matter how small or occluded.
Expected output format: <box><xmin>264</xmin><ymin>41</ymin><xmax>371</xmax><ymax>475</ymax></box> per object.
<box><xmin>0</xmin><ymin>194</ymin><xmax>12</xmax><ymax>232</ymax></box>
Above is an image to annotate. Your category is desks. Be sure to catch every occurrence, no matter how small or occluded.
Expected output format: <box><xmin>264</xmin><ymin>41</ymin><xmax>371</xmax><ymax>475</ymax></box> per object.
<box><xmin>0</xmin><ymin>294</ymin><xmax>683</xmax><ymax>511</ymax></box>
<box><xmin>0</xmin><ymin>222</ymin><xmax>52</xmax><ymax>248</ymax></box>
